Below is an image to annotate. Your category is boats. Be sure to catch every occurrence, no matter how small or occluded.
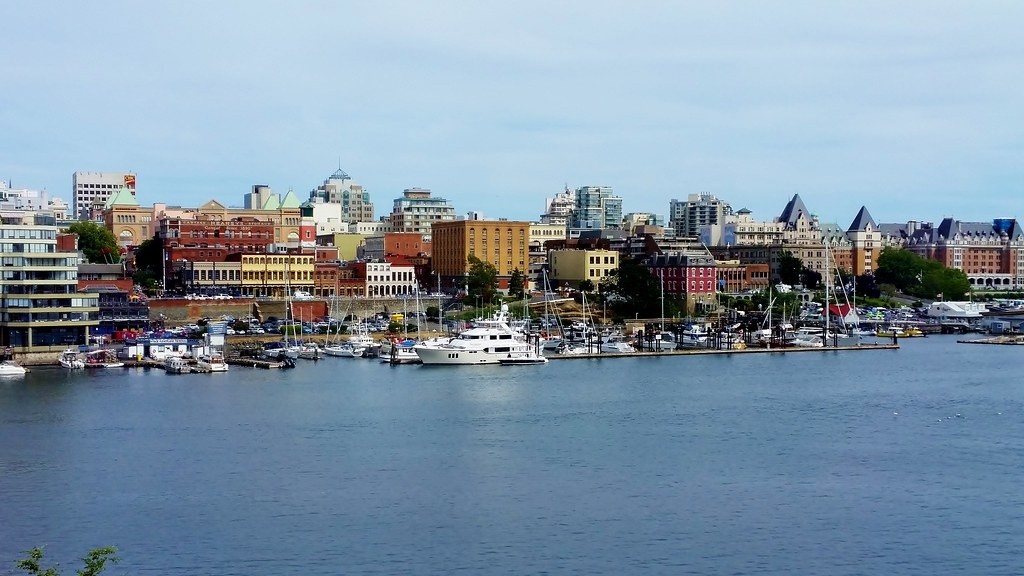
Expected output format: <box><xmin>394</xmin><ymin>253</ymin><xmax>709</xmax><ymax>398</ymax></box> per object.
<box><xmin>0</xmin><ymin>360</ymin><xmax>26</xmax><ymax>375</ymax></box>
<box><xmin>412</xmin><ymin>306</ymin><xmax>549</xmax><ymax>366</ymax></box>
<box><xmin>57</xmin><ymin>241</ymin><xmax>1024</xmax><ymax>375</ymax></box>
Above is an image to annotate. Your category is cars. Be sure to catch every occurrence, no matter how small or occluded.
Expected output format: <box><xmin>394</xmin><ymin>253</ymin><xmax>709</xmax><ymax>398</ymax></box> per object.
<box><xmin>193</xmin><ymin>293</ymin><xmax>214</xmax><ymax>301</ymax></box>
<box><xmin>213</xmin><ymin>293</ymin><xmax>234</xmax><ymax>301</ymax></box>
